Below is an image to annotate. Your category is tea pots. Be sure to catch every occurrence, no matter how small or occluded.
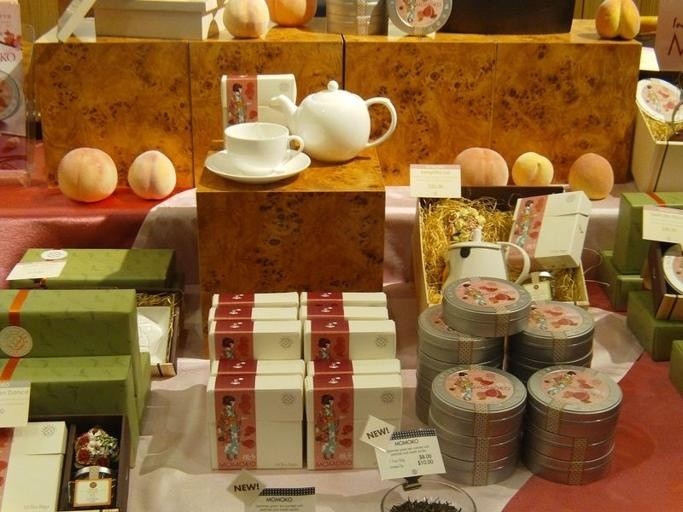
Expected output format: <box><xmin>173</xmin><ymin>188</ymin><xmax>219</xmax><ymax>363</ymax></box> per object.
<box><xmin>267</xmin><ymin>79</ymin><xmax>399</xmax><ymax>163</ymax></box>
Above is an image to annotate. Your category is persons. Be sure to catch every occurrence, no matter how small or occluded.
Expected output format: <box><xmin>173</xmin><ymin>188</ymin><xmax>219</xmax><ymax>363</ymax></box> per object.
<box><xmin>547</xmin><ymin>370</ymin><xmax>576</xmax><ymax>396</ymax></box>
<box><xmin>530</xmin><ymin>307</ymin><xmax>547</xmax><ymax>332</ymax></box>
<box><xmin>462</xmin><ymin>282</ymin><xmax>489</xmax><ymax>306</ymax></box>
<box><xmin>220</xmin><ymin>337</ymin><xmax>236</xmax><ymax>359</ymax></box>
<box><xmin>221</xmin><ymin>395</ymin><xmax>242</xmax><ymax>461</ymax></box>
<box><xmin>228</xmin><ymin>83</ymin><xmax>246</xmax><ymax>124</ymax></box>
<box><xmin>646</xmin><ymin>85</ymin><xmax>661</xmax><ymax>113</ymax></box>
<box><xmin>317</xmin><ymin>394</ymin><xmax>338</xmax><ymax>458</ymax></box>
<box><xmin>317</xmin><ymin>337</ymin><xmax>331</xmax><ymax>361</ymax></box>
<box><xmin>454</xmin><ymin>372</ymin><xmax>473</xmax><ymax>401</ymax></box>
<box><xmin>514</xmin><ymin>200</ymin><xmax>534</xmax><ymax>247</ymax></box>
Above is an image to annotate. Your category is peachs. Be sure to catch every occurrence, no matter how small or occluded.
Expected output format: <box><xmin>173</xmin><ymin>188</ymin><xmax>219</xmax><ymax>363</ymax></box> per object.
<box><xmin>223</xmin><ymin>0</ymin><xmax>270</xmax><ymax>39</ymax></box>
<box><xmin>568</xmin><ymin>151</ymin><xmax>615</xmax><ymax>199</ymax></box>
<box><xmin>512</xmin><ymin>151</ymin><xmax>554</xmax><ymax>188</ymax></box>
<box><xmin>452</xmin><ymin>146</ymin><xmax>511</xmax><ymax>186</ymax></box>
<box><xmin>57</xmin><ymin>146</ymin><xmax>118</xmax><ymax>204</ymax></box>
<box><xmin>595</xmin><ymin>0</ymin><xmax>641</xmax><ymax>40</ymax></box>
<box><xmin>266</xmin><ymin>0</ymin><xmax>317</xmax><ymax>27</ymax></box>
<box><xmin>128</xmin><ymin>149</ymin><xmax>177</xmax><ymax>201</ymax></box>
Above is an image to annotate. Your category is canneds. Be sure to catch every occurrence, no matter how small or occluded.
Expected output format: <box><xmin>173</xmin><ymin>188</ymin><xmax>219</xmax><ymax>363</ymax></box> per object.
<box><xmin>71</xmin><ymin>467</ymin><xmax>114</xmax><ymax>508</ymax></box>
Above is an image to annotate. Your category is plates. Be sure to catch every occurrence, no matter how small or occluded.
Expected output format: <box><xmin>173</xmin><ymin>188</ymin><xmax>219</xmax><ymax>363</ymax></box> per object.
<box><xmin>203</xmin><ymin>148</ymin><xmax>312</xmax><ymax>185</ymax></box>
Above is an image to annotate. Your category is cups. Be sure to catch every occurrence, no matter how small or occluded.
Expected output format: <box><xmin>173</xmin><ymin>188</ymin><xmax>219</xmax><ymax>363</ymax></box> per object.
<box><xmin>222</xmin><ymin>120</ymin><xmax>303</xmax><ymax>175</ymax></box>
<box><xmin>380</xmin><ymin>480</ymin><xmax>477</xmax><ymax>512</ymax></box>
<box><xmin>440</xmin><ymin>227</ymin><xmax>531</xmax><ymax>309</ymax></box>
